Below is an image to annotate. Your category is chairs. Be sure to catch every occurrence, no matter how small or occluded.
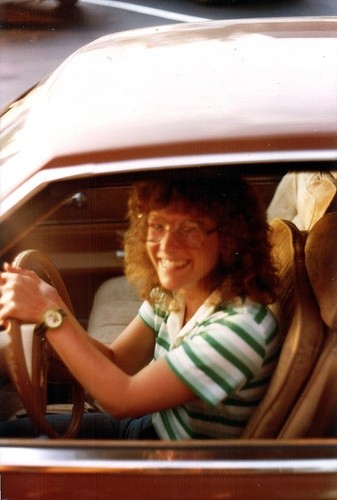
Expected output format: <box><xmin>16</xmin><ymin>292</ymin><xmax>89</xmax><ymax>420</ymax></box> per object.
<box><xmin>87</xmin><ymin>171</ymin><xmax>337</xmax><ymax>441</ymax></box>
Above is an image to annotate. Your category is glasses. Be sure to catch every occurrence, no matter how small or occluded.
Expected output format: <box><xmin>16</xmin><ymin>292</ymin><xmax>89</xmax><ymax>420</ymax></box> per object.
<box><xmin>137</xmin><ymin>214</ymin><xmax>218</xmax><ymax>249</ymax></box>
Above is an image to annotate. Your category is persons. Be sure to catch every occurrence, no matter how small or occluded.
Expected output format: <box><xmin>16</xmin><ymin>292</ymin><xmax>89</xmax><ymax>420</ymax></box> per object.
<box><xmin>0</xmin><ymin>170</ymin><xmax>284</xmax><ymax>442</ymax></box>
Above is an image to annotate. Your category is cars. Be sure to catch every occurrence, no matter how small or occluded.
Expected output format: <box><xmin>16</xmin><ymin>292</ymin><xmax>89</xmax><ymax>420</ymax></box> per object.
<box><xmin>1</xmin><ymin>14</ymin><xmax>337</xmax><ymax>500</ymax></box>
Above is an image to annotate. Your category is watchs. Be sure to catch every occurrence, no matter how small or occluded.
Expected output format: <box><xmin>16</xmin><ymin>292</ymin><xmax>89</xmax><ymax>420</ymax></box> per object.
<box><xmin>34</xmin><ymin>308</ymin><xmax>69</xmax><ymax>336</ymax></box>
